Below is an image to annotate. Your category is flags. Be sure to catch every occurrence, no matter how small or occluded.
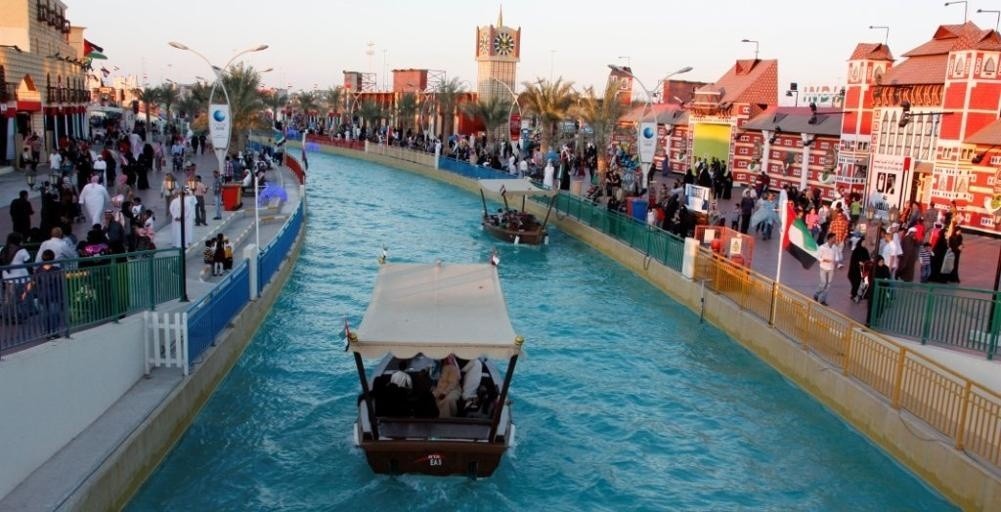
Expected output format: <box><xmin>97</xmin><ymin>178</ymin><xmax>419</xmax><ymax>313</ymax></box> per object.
<box><xmin>782</xmin><ymin>198</ymin><xmax>824</xmax><ymax>262</ymax></box>
<box><xmin>84</xmin><ymin>39</ymin><xmax>108</xmax><ymax>61</ymax></box>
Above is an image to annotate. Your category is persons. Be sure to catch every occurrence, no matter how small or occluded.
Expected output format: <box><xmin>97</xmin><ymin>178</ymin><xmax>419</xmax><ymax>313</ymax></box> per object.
<box><xmin>308</xmin><ymin>123</ymin><xmax>459</xmax><ymax>159</ymax></box>
<box><xmin>587</xmin><ymin>155</ymin><xmax>965</xmax><ymax>327</ymax></box>
<box><xmin>490</xmin><ymin>209</ymin><xmax>540</xmax><ymax>233</ymax></box>
<box><xmin>458</xmin><ymin>134</ymin><xmax>598</xmax><ymax>192</ymax></box>
<box><xmin>203</xmin><ymin>233</ymin><xmax>234</xmax><ymax>277</ymax></box>
<box><xmin>222</xmin><ymin>137</ymin><xmax>285</xmax><ymax>186</ymax></box>
<box><xmin>390</xmin><ymin>359</ymin><xmax>413</xmax><ymax>391</ymax></box>
<box><xmin>194</xmin><ymin>175</ymin><xmax>209</xmax><ymax>226</ymax></box>
<box><xmin>150</xmin><ymin>122</ymin><xmax>208</xmax><ymax>173</ymax></box>
<box><xmin>212</xmin><ymin>171</ymin><xmax>223</xmax><ymax>220</ymax></box>
<box><xmin>0</xmin><ymin>122</ymin><xmax>155</xmax><ymax>340</ymax></box>
<box><xmin>432</xmin><ymin>356</ymin><xmax>482</xmax><ymax>419</ymax></box>
<box><xmin>160</xmin><ymin>173</ymin><xmax>179</xmax><ymax>215</ymax></box>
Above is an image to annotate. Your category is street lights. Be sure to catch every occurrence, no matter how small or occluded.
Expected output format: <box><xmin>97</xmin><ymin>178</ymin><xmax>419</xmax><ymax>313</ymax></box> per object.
<box><xmin>944</xmin><ymin>0</ymin><xmax>967</xmax><ymax>24</ymax></box>
<box><xmin>168</xmin><ymin>41</ymin><xmax>270</xmax><ymax>187</ymax></box>
<box><xmin>868</xmin><ymin>24</ymin><xmax>888</xmax><ymax>45</ymax></box>
<box><xmin>165</xmin><ymin>172</ymin><xmax>198</xmax><ymax>303</ymax></box>
<box><xmin>486</xmin><ymin>75</ymin><xmax>546</xmax><ymax>152</ymax></box>
<box><xmin>23</xmin><ymin>167</ymin><xmax>62</xmax><ymax>234</ymax></box>
<box><xmin>607</xmin><ymin>64</ymin><xmax>693</xmax><ymax>192</ymax></box>
<box><xmin>741</xmin><ymin>39</ymin><xmax>758</xmax><ymax>59</ymax></box>
<box><xmin>977</xmin><ymin>8</ymin><xmax>1000</xmax><ymax>31</ymax></box>
<box><xmin>785</xmin><ymin>90</ymin><xmax>798</xmax><ymax>106</ymax></box>
<box><xmin>336</xmin><ymin>84</ymin><xmax>373</xmax><ymax>129</ymax></box>
<box><xmin>407</xmin><ymin>82</ymin><xmax>441</xmax><ymax>144</ymax></box>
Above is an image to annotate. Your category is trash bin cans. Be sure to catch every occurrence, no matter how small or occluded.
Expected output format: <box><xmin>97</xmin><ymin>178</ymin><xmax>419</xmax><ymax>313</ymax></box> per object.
<box><xmin>222</xmin><ymin>184</ymin><xmax>243</xmax><ymax>211</ymax></box>
<box><xmin>572</xmin><ymin>181</ymin><xmax>583</xmax><ymax>195</ymax></box>
<box><xmin>627</xmin><ymin>197</ymin><xmax>649</xmax><ymax>222</ymax></box>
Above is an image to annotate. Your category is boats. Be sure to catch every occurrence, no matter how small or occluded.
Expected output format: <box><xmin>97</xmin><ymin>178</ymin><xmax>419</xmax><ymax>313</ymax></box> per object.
<box><xmin>474</xmin><ymin>174</ymin><xmax>559</xmax><ymax>246</ymax></box>
<box><xmin>342</xmin><ymin>251</ymin><xmax>523</xmax><ymax>480</ymax></box>
